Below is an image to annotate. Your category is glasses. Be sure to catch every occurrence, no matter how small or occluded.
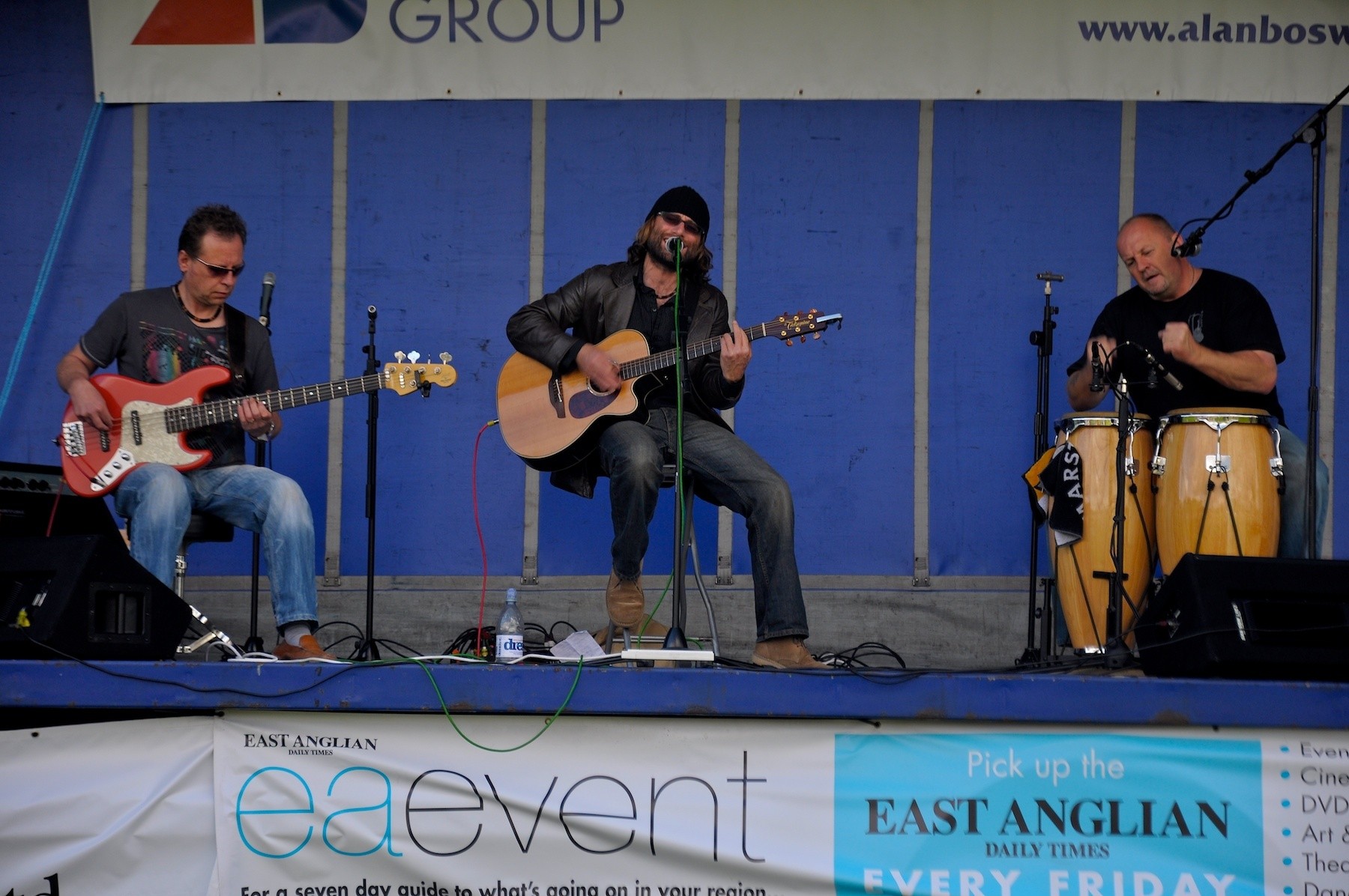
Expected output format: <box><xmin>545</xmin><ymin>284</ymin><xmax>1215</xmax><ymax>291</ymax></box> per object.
<box><xmin>656</xmin><ymin>211</ymin><xmax>707</xmax><ymax>236</ymax></box>
<box><xmin>193</xmin><ymin>254</ymin><xmax>245</xmax><ymax>279</ymax></box>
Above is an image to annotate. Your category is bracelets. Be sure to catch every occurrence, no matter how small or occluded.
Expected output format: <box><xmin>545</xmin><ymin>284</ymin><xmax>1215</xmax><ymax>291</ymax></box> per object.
<box><xmin>249</xmin><ymin>420</ymin><xmax>277</xmax><ymax>440</ymax></box>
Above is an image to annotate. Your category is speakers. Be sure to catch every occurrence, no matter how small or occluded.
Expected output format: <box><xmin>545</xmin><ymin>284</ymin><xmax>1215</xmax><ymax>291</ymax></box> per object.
<box><xmin>1134</xmin><ymin>552</ymin><xmax>1349</xmax><ymax>684</ymax></box>
<box><xmin>0</xmin><ymin>461</ymin><xmax>193</xmax><ymax>662</ymax></box>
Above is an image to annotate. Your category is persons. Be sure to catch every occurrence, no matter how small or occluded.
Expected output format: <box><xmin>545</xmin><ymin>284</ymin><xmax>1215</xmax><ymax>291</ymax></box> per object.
<box><xmin>507</xmin><ymin>185</ymin><xmax>835</xmax><ymax>669</ymax></box>
<box><xmin>59</xmin><ymin>203</ymin><xmax>337</xmax><ymax>663</ymax></box>
<box><xmin>1067</xmin><ymin>213</ymin><xmax>1330</xmax><ymax>658</ymax></box>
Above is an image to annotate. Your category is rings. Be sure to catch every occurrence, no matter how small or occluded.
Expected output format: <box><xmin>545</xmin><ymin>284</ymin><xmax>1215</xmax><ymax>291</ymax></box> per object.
<box><xmin>249</xmin><ymin>418</ymin><xmax>256</xmax><ymax>424</ymax></box>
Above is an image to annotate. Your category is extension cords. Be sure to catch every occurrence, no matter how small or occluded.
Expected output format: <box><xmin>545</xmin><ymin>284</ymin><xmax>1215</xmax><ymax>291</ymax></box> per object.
<box><xmin>619</xmin><ymin>649</ymin><xmax>714</xmax><ymax>661</ymax></box>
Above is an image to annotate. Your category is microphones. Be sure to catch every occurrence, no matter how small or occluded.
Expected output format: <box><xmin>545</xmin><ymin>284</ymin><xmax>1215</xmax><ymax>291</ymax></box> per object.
<box><xmin>1143</xmin><ymin>351</ymin><xmax>1183</xmax><ymax>390</ymax></box>
<box><xmin>665</xmin><ymin>236</ymin><xmax>684</xmax><ymax>253</ymax></box>
<box><xmin>258</xmin><ymin>272</ymin><xmax>278</xmax><ymax>327</ymax></box>
<box><xmin>1171</xmin><ymin>243</ymin><xmax>1202</xmax><ymax>258</ymax></box>
<box><xmin>1092</xmin><ymin>342</ymin><xmax>1104</xmax><ymax>378</ymax></box>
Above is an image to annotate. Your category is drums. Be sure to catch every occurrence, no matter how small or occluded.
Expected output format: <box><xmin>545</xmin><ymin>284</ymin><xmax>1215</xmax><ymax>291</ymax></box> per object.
<box><xmin>1154</xmin><ymin>407</ymin><xmax>1281</xmax><ymax>577</ymax></box>
<box><xmin>1048</xmin><ymin>411</ymin><xmax>1156</xmax><ymax>657</ymax></box>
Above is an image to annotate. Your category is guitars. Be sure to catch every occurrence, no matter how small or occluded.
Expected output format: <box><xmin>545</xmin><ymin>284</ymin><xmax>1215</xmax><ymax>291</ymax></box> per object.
<box><xmin>60</xmin><ymin>351</ymin><xmax>457</xmax><ymax>497</ymax></box>
<box><xmin>496</xmin><ymin>308</ymin><xmax>828</xmax><ymax>469</ymax></box>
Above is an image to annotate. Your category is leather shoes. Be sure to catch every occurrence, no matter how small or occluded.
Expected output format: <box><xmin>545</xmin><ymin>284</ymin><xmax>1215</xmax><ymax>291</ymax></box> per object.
<box><xmin>271</xmin><ymin>636</ymin><xmax>339</xmax><ymax>660</ymax></box>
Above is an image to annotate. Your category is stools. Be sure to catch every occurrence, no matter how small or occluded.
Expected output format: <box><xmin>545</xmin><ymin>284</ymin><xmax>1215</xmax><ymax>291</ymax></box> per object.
<box><xmin>603</xmin><ymin>465</ymin><xmax>723</xmax><ymax>670</ymax></box>
<box><xmin>126</xmin><ymin>506</ymin><xmax>235</xmax><ymax>661</ymax></box>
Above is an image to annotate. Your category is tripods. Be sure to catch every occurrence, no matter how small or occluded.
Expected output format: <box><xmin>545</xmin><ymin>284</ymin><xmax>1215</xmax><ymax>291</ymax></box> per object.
<box><xmin>567</xmin><ymin>249</ymin><xmax>778</xmax><ymax>672</ymax></box>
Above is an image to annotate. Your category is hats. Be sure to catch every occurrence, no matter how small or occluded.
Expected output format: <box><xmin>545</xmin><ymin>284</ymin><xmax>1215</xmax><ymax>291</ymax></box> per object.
<box><xmin>645</xmin><ymin>185</ymin><xmax>712</xmax><ymax>234</ymax></box>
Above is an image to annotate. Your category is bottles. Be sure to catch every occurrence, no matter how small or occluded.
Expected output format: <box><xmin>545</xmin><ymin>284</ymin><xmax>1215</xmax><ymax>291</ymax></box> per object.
<box><xmin>493</xmin><ymin>586</ymin><xmax>525</xmax><ymax>666</ymax></box>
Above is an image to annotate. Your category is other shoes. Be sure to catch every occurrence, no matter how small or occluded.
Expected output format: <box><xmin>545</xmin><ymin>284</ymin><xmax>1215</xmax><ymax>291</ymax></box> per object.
<box><xmin>604</xmin><ymin>557</ymin><xmax>649</xmax><ymax>626</ymax></box>
<box><xmin>750</xmin><ymin>634</ymin><xmax>836</xmax><ymax>670</ymax></box>
